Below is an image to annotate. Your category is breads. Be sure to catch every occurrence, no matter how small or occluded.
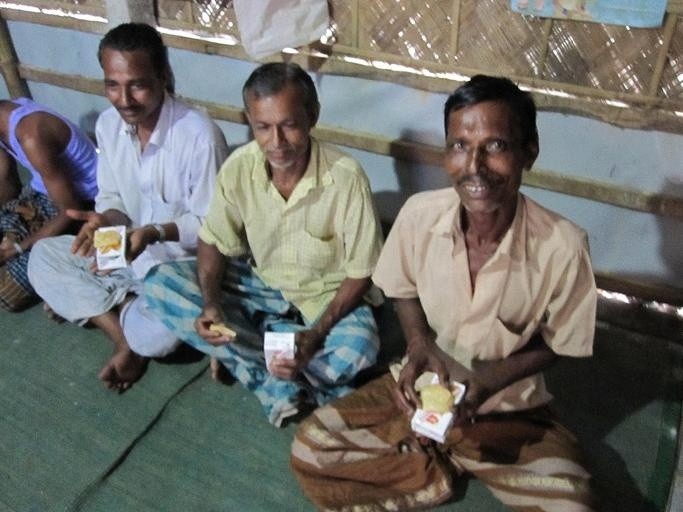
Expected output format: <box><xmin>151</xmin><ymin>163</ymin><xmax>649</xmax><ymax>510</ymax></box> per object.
<box><xmin>92</xmin><ymin>230</ymin><xmax>121</xmax><ymax>252</ymax></box>
<box><xmin>416</xmin><ymin>386</ymin><xmax>453</xmax><ymax>414</ymax></box>
<box><xmin>207</xmin><ymin>323</ymin><xmax>237</xmax><ymax>339</ymax></box>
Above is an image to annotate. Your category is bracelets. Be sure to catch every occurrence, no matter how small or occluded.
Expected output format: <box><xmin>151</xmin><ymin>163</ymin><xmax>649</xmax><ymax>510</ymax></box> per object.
<box><xmin>152</xmin><ymin>222</ymin><xmax>166</xmax><ymax>241</ymax></box>
<box><xmin>405</xmin><ymin>336</ymin><xmax>429</xmax><ymax>356</ymax></box>
<box><xmin>14</xmin><ymin>242</ymin><xmax>23</xmax><ymax>255</ymax></box>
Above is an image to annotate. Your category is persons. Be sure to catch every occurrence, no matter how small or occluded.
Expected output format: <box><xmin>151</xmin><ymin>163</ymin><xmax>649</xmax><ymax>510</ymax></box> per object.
<box><xmin>141</xmin><ymin>63</ymin><xmax>384</xmax><ymax>430</ymax></box>
<box><xmin>290</xmin><ymin>74</ymin><xmax>598</xmax><ymax>512</ymax></box>
<box><xmin>1</xmin><ymin>95</ymin><xmax>98</xmax><ymax>312</ymax></box>
<box><xmin>26</xmin><ymin>22</ymin><xmax>232</xmax><ymax>395</ymax></box>
